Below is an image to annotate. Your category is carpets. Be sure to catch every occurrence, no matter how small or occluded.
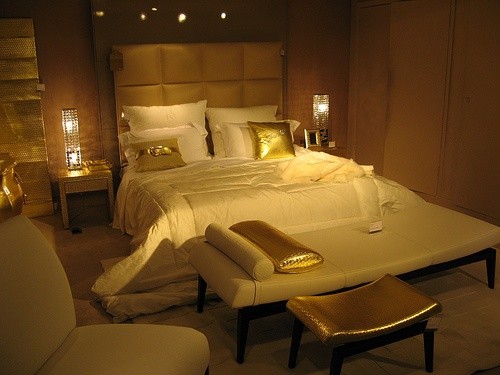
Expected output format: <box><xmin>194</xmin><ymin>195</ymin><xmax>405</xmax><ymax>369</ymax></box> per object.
<box><xmin>100</xmin><ymin>244</ymin><xmax>500</xmax><ymax>375</ymax></box>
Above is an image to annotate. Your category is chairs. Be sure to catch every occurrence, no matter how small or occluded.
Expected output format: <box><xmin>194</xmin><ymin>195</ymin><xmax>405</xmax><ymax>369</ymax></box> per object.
<box><xmin>0</xmin><ymin>162</ymin><xmax>28</xmax><ymax>220</ymax></box>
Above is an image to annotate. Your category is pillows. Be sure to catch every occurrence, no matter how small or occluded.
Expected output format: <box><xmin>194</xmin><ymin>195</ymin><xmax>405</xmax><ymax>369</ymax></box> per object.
<box><xmin>206</xmin><ymin>103</ymin><xmax>280</xmax><ymax>159</ymax></box>
<box><xmin>214</xmin><ymin>120</ymin><xmax>301</xmax><ymax>159</ymax></box>
<box><xmin>122</xmin><ymin>100</ymin><xmax>212</xmax><ymax>161</ymax></box>
<box><xmin>131</xmin><ymin>138</ymin><xmax>187</xmax><ymax>172</ymax></box>
<box><xmin>118</xmin><ymin>121</ymin><xmax>208</xmax><ymax>168</ymax></box>
<box><xmin>247</xmin><ymin>120</ymin><xmax>296</xmax><ymax>159</ymax></box>
<box><xmin>228</xmin><ymin>220</ymin><xmax>323</xmax><ymax>273</ymax></box>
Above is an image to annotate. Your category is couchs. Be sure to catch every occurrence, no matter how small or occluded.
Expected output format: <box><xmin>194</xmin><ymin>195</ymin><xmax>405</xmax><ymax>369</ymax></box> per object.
<box><xmin>0</xmin><ymin>209</ymin><xmax>210</xmax><ymax>375</ymax></box>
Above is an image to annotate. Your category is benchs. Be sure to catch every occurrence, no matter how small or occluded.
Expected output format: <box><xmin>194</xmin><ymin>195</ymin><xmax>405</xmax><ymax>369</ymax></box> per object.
<box><xmin>187</xmin><ymin>202</ymin><xmax>500</xmax><ymax>364</ymax></box>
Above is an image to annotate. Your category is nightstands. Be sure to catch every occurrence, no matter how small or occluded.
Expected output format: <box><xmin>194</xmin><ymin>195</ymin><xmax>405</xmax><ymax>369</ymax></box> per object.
<box><xmin>297</xmin><ymin>142</ymin><xmax>347</xmax><ymax>159</ymax></box>
<box><xmin>57</xmin><ymin>166</ymin><xmax>115</xmax><ymax>230</ymax></box>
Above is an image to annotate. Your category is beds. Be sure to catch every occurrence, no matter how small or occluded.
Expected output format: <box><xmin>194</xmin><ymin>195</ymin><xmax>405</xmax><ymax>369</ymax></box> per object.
<box><xmin>94</xmin><ymin>40</ymin><xmax>427</xmax><ymax>298</ymax></box>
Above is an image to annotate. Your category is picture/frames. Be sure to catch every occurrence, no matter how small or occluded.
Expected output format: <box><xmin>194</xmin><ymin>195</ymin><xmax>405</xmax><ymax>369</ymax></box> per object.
<box><xmin>304</xmin><ymin>128</ymin><xmax>321</xmax><ymax>148</ymax></box>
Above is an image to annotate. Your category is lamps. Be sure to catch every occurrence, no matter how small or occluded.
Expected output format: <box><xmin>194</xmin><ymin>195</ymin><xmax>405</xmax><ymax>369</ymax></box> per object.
<box><xmin>313</xmin><ymin>94</ymin><xmax>330</xmax><ymax>146</ymax></box>
<box><xmin>61</xmin><ymin>108</ymin><xmax>82</xmax><ymax>170</ymax></box>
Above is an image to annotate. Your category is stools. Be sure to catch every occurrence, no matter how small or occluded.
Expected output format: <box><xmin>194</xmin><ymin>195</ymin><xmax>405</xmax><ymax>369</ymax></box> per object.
<box><xmin>286</xmin><ymin>273</ymin><xmax>441</xmax><ymax>375</ymax></box>
